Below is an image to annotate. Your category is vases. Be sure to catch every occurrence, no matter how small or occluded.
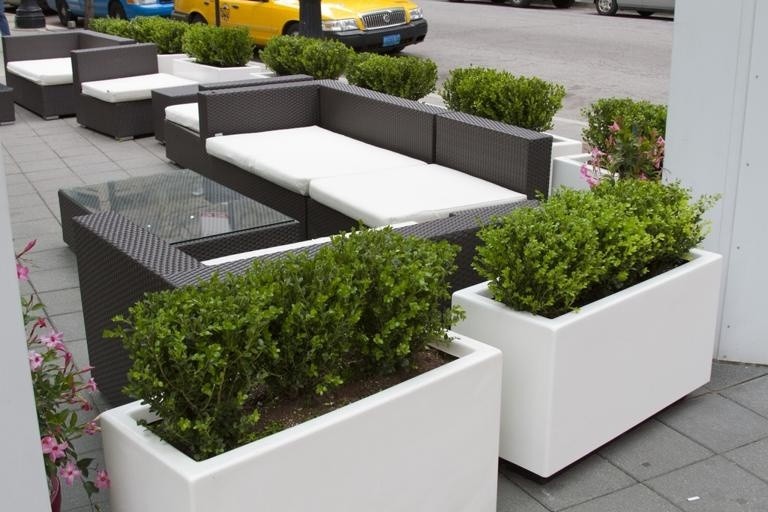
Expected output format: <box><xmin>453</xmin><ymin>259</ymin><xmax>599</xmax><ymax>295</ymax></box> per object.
<box><xmin>45</xmin><ymin>469</ymin><xmax>61</xmax><ymax>512</ymax></box>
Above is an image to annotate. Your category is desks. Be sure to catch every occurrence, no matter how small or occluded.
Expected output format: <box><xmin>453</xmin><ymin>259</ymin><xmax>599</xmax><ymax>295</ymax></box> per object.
<box><xmin>58</xmin><ymin>169</ymin><xmax>304</xmax><ymax>262</ymax></box>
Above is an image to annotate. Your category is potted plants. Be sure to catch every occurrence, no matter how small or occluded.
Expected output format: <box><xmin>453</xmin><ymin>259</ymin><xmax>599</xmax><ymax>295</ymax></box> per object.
<box><xmin>551</xmin><ymin>95</ymin><xmax>667</xmax><ymax>195</ymax></box>
<box><xmin>452</xmin><ymin>174</ymin><xmax>724</xmax><ymax>481</ymax></box>
<box><xmin>98</xmin><ymin>215</ymin><xmax>502</xmax><ymax>512</ymax></box>
<box><xmin>439</xmin><ymin>65</ymin><xmax>581</xmax><ymax>197</ymax></box>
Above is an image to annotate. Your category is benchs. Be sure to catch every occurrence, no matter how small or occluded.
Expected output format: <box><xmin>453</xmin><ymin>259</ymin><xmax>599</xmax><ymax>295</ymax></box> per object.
<box><xmin>68</xmin><ymin>198</ymin><xmax>541</xmax><ymax>409</ymax></box>
<box><xmin>198</xmin><ymin>78</ymin><xmax>554</xmax><ymax>240</ymax></box>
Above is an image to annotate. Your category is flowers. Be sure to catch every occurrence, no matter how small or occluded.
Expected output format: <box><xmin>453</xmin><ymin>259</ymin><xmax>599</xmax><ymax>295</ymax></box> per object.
<box><xmin>12</xmin><ymin>238</ymin><xmax>111</xmax><ymax>512</ymax></box>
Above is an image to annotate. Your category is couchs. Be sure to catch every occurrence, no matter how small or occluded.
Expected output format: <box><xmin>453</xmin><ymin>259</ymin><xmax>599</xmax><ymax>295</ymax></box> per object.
<box><xmin>2</xmin><ymin>29</ymin><xmax>314</xmax><ymax>168</ymax></box>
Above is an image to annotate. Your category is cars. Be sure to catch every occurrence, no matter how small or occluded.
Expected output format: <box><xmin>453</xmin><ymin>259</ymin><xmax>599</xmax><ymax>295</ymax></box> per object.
<box><xmin>449</xmin><ymin>1</ymin><xmax>577</xmax><ymax>9</ymax></box>
<box><xmin>2</xmin><ymin>1</ymin><xmax>54</xmax><ymax>17</ymax></box>
<box><xmin>171</xmin><ymin>1</ymin><xmax>428</xmax><ymax>55</ymax></box>
<box><xmin>594</xmin><ymin>1</ymin><xmax>675</xmax><ymax>17</ymax></box>
<box><xmin>47</xmin><ymin>1</ymin><xmax>175</xmax><ymax>28</ymax></box>
